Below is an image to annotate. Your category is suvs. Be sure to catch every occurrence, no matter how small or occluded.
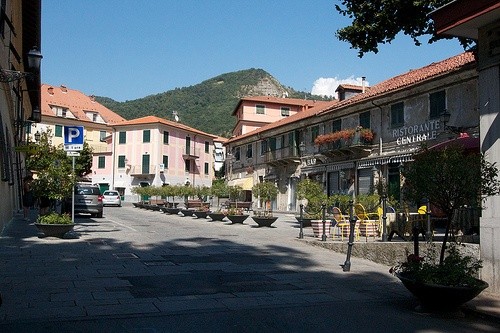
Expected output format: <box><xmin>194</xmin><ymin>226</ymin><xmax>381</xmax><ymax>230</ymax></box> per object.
<box><xmin>66</xmin><ymin>184</ymin><xmax>104</xmax><ymax>218</ymax></box>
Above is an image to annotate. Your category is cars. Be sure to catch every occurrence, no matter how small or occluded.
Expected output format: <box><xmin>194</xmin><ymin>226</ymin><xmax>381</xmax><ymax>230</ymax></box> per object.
<box><xmin>102</xmin><ymin>190</ymin><xmax>121</xmax><ymax>206</ymax></box>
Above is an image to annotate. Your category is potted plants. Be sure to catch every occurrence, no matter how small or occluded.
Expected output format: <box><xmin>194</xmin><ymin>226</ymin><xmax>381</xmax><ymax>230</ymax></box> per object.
<box><xmin>396</xmin><ymin>141</ymin><xmax>499</xmax><ymax>306</ymax></box>
<box><xmin>131</xmin><ymin>183</ymin><xmax>278</xmax><ymax>228</ymax></box>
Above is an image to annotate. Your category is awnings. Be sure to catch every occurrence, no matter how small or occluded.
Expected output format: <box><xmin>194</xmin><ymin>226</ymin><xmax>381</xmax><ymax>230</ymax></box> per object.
<box><xmin>413</xmin><ymin>131</ymin><xmax>480</xmax><ymax>159</ymax></box>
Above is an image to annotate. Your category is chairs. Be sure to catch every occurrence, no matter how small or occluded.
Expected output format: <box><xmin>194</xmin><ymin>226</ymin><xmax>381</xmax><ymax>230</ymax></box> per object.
<box><xmin>331</xmin><ymin>206</ymin><xmax>359</xmax><ymax>241</ymax></box>
<box><xmin>354</xmin><ymin>203</ymin><xmax>379</xmax><ymax>241</ymax></box>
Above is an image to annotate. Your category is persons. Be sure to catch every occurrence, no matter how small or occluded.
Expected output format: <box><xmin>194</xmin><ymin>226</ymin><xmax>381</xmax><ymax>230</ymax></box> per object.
<box><xmin>21</xmin><ymin>174</ymin><xmax>52</xmax><ymax>220</ymax></box>
